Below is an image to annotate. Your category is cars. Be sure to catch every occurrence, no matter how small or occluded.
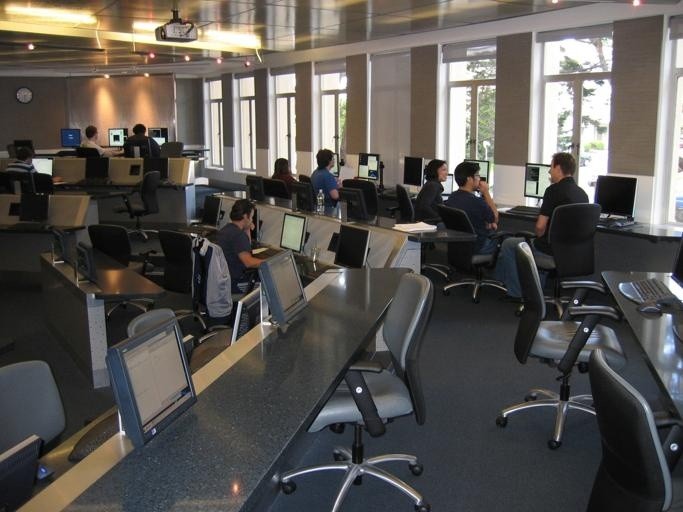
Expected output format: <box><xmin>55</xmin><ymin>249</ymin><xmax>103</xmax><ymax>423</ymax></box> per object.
<box><xmin>567</xmin><ymin>150</ymin><xmax>593</xmax><ymax>168</ymax></box>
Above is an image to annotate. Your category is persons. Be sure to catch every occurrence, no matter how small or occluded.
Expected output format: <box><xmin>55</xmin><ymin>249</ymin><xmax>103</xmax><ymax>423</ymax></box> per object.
<box><xmin>494</xmin><ymin>152</ymin><xmax>589</xmax><ymax>297</ymax></box>
<box><xmin>79</xmin><ymin>126</ymin><xmax>112</xmax><ymax>156</ymax></box>
<box><xmin>122</xmin><ymin>125</ymin><xmax>159</xmax><ymax>158</ymax></box>
<box><xmin>271</xmin><ymin>158</ymin><xmax>299</xmax><ymax>199</ymax></box>
<box><xmin>445</xmin><ymin>162</ymin><xmax>511</xmax><ymax>255</ymax></box>
<box><xmin>4</xmin><ymin>148</ymin><xmax>63</xmax><ymax>184</ymax></box>
<box><xmin>310</xmin><ymin>149</ymin><xmax>342</xmax><ymax>208</ymax></box>
<box><xmin>413</xmin><ymin>159</ymin><xmax>447</xmax><ymax>225</ymax></box>
<box><xmin>214</xmin><ymin>200</ymin><xmax>266</xmax><ymax>294</ymax></box>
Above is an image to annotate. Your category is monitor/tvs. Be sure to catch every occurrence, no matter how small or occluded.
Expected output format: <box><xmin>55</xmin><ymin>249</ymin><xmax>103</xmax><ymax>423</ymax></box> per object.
<box><xmin>404</xmin><ymin>156</ymin><xmax>425</xmax><ymax>188</ymax></box>
<box><xmin>524</xmin><ymin>163</ymin><xmax>552</xmax><ymax>200</ymax></box>
<box><xmin>280</xmin><ymin>213</ymin><xmax>311</xmax><ymax>254</ymax></box>
<box><xmin>33</xmin><ymin>158</ymin><xmax>53</xmax><ymax>176</ymax></box>
<box><xmin>230</xmin><ymin>287</ymin><xmax>261</xmax><ymax>346</ymax></box>
<box><xmin>108</xmin><ymin>128</ymin><xmax>125</xmax><ymax>147</ymax></box>
<box><xmin>671</xmin><ymin>232</ymin><xmax>683</xmax><ymax>342</ymax></box>
<box><xmin>594</xmin><ymin>176</ymin><xmax>638</xmax><ymax>218</ymax></box>
<box><xmin>258</xmin><ymin>250</ymin><xmax>308</xmax><ymax>326</ymax></box>
<box><xmin>152</xmin><ymin>137</ymin><xmax>166</xmax><ymax>150</ymax></box>
<box><xmin>358</xmin><ymin>153</ymin><xmax>380</xmax><ymax>181</ymax></box>
<box><xmin>105</xmin><ymin>317</ymin><xmax>197</xmax><ymax>449</ymax></box>
<box><xmin>329</xmin><ymin>154</ymin><xmax>341</xmax><ymax>178</ymax></box>
<box><xmin>202</xmin><ymin>196</ymin><xmax>224</xmax><ymax>228</ymax></box>
<box><xmin>334</xmin><ymin>224</ymin><xmax>372</xmax><ymax>271</ymax></box>
<box><xmin>463</xmin><ymin>159</ymin><xmax>490</xmax><ymax>192</ymax></box>
<box><xmin>61</xmin><ymin>128</ymin><xmax>81</xmax><ymax>148</ymax></box>
<box><xmin>148</xmin><ymin>128</ymin><xmax>168</xmax><ymax>142</ymax></box>
<box><xmin>250</xmin><ymin>209</ymin><xmax>264</xmax><ymax>245</ymax></box>
<box><xmin>0</xmin><ymin>435</ymin><xmax>41</xmax><ymax>512</ymax></box>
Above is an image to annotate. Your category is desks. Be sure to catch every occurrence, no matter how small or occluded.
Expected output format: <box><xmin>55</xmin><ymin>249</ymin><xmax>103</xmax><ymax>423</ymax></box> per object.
<box><xmin>14</xmin><ymin>265</ymin><xmax>414</xmax><ymax>512</ymax></box>
<box><xmin>594</xmin><ymin>267</ymin><xmax>682</xmax><ymax>427</ymax></box>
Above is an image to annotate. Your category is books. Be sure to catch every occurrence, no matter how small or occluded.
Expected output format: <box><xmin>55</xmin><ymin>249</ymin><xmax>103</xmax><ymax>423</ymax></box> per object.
<box><xmin>391</xmin><ymin>221</ymin><xmax>437</xmax><ymax>233</ymax></box>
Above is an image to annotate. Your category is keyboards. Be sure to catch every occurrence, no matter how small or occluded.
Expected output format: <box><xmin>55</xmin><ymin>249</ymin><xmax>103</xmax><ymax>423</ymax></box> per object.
<box><xmin>507</xmin><ymin>207</ymin><xmax>543</xmax><ymax>217</ymax></box>
<box><xmin>253</xmin><ymin>249</ymin><xmax>307</xmax><ymax>270</ymax></box>
<box><xmin>618</xmin><ymin>278</ymin><xmax>681</xmax><ymax>306</ymax></box>
<box><xmin>597</xmin><ymin>219</ymin><xmax>628</xmax><ymax>228</ymax></box>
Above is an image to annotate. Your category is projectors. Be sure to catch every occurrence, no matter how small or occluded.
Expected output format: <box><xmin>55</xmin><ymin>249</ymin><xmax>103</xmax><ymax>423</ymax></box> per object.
<box><xmin>155</xmin><ymin>22</ymin><xmax>198</xmax><ymax>43</ymax></box>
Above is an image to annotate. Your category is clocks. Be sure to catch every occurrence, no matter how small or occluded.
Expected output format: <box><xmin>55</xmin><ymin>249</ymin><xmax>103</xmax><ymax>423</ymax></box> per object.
<box><xmin>15</xmin><ymin>86</ymin><xmax>33</xmax><ymax>104</ymax></box>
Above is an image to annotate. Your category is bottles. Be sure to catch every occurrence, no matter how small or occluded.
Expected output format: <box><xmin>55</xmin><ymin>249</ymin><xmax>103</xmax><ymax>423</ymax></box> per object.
<box><xmin>315</xmin><ymin>189</ymin><xmax>324</xmax><ymax>213</ymax></box>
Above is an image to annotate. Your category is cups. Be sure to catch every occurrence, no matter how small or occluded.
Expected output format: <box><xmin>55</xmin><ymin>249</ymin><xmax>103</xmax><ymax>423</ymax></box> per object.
<box><xmin>310</xmin><ymin>248</ymin><xmax>320</xmax><ymax>262</ymax></box>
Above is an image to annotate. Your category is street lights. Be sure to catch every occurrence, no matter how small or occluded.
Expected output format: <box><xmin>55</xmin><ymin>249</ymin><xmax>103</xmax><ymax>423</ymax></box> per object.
<box><xmin>482</xmin><ymin>141</ymin><xmax>491</xmax><ymax>162</ymax></box>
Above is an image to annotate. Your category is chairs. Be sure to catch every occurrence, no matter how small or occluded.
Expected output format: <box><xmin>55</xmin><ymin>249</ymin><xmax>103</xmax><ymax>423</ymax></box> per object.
<box><xmin>492</xmin><ymin>241</ymin><xmax>628</xmax><ymax>451</ymax></box>
<box><xmin>0</xmin><ymin>359</ymin><xmax>68</xmax><ymax>461</ymax></box>
<box><xmin>583</xmin><ymin>346</ymin><xmax>682</xmax><ymax>512</ymax></box>
<box><xmin>276</xmin><ymin>271</ymin><xmax>437</xmax><ymax>512</ymax></box>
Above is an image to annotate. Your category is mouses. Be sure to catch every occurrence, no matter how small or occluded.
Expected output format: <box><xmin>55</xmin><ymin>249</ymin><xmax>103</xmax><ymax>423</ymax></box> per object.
<box><xmin>36</xmin><ymin>463</ymin><xmax>54</xmax><ymax>481</ymax></box>
<box><xmin>637</xmin><ymin>302</ymin><xmax>662</xmax><ymax>312</ymax></box>
